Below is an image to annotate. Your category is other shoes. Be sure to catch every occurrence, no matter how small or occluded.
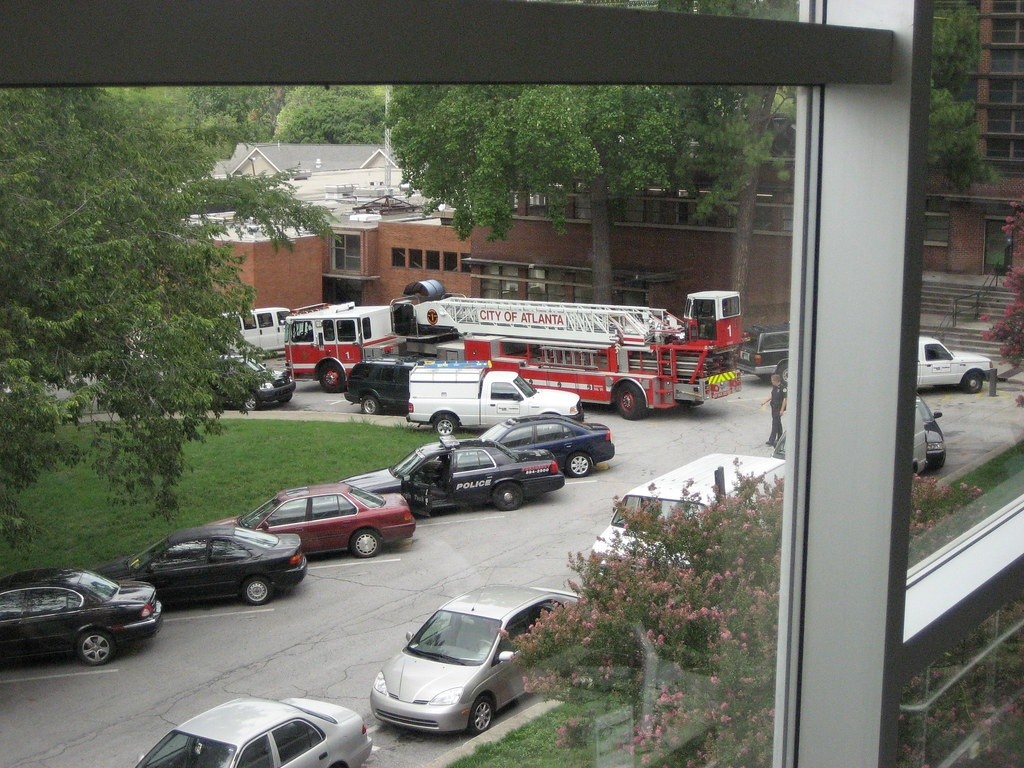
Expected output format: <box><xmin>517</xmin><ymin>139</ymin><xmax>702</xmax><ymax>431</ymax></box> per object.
<box><xmin>766</xmin><ymin>441</ymin><xmax>774</xmax><ymax>444</ymax></box>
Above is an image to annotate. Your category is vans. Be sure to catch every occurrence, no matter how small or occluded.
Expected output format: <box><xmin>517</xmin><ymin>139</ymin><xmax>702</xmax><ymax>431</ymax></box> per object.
<box><xmin>587</xmin><ymin>452</ymin><xmax>785</xmax><ymax>584</ymax></box>
<box><xmin>771</xmin><ymin>398</ymin><xmax>925</xmax><ymax>475</ymax></box>
<box><xmin>218</xmin><ymin>307</ymin><xmax>290</xmax><ymax>357</ymax></box>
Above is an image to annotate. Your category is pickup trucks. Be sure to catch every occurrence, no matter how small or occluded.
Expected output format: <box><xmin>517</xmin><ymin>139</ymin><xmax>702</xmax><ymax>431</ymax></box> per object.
<box><xmin>736</xmin><ymin>331</ymin><xmax>789</xmax><ymax>385</ymax></box>
<box><xmin>916</xmin><ymin>337</ymin><xmax>993</xmax><ymax>393</ymax></box>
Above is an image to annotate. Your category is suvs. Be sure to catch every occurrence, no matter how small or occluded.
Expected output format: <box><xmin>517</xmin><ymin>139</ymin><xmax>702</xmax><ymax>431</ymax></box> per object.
<box><xmin>207</xmin><ymin>355</ymin><xmax>296</xmax><ymax>411</ymax></box>
<box><xmin>344</xmin><ymin>354</ymin><xmax>423</xmax><ymax>415</ymax></box>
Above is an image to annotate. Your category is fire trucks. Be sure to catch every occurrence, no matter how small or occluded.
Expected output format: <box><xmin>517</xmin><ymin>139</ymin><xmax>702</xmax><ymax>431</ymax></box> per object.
<box><xmin>277</xmin><ymin>274</ymin><xmax>752</xmax><ymax>420</ymax></box>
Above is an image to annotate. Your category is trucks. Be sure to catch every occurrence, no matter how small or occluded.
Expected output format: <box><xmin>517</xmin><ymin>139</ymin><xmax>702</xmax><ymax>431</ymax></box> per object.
<box><xmin>406</xmin><ymin>359</ymin><xmax>585</xmax><ymax>436</ymax></box>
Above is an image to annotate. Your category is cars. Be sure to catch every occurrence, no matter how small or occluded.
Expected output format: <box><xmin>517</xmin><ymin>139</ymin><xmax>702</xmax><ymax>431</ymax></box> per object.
<box><xmin>206</xmin><ymin>481</ymin><xmax>417</xmax><ymax>559</ymax></box>
<box><xmin>95</xmin><ymin>526</ymin><xmax>308</xmax><ymax>607</ymax></box>
<box><xmin>339</xmin><ymin>437</ymin><xmax>565</xmax><ymax>517</ymax></box>
<box><xmin>915</xmin><ymin>396</ymin><xmax>946</xmax><ymax>472</ymax></box>
<box><xmin>0</xmin><ymin>567</ymin><xmax>163</xmax><ymax>666</ymax></box>
<box><xmin>370</xmin><ymin>584</ymin><xmax>583</xmax><ymax>736</ymax></box>
<box><xmin>133</xmin><ymin>696</ymin><xmax>373</xmax><ymax>768</ymax></box>
<box><xmin>479</xmin><ymin>413</ymin><xmax>615</xmax><ymax>478</ymax></box>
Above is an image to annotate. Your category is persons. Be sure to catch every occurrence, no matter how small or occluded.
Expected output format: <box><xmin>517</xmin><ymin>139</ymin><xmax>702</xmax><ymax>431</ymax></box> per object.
<box><xmin>760</xmin><ymin>374</ymin><xmax>787</xmax><ymax>444</ymax></box>
